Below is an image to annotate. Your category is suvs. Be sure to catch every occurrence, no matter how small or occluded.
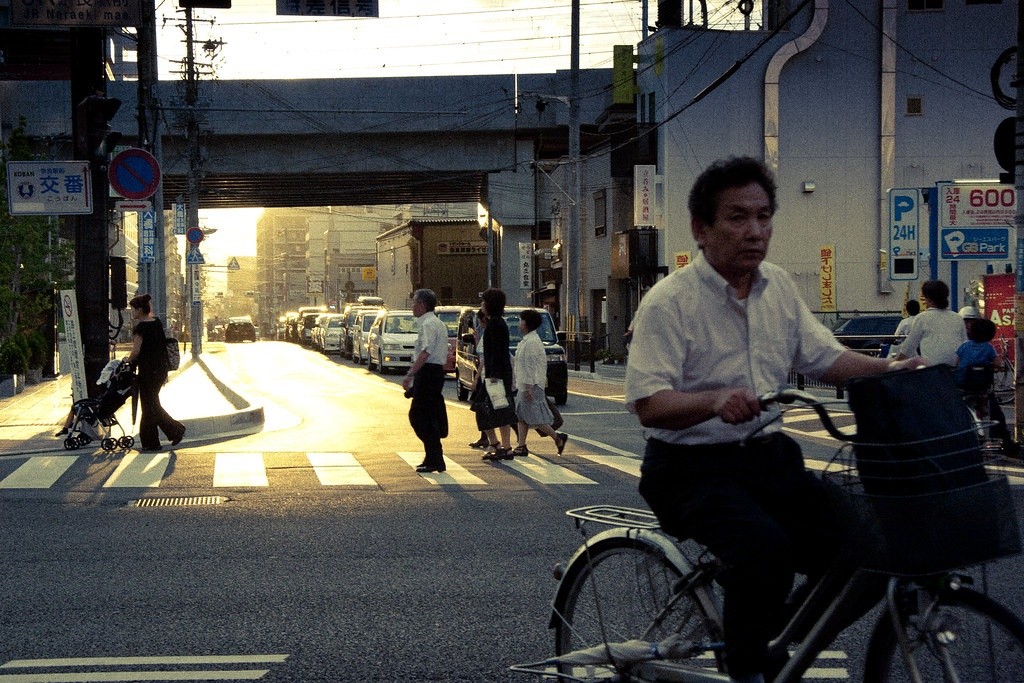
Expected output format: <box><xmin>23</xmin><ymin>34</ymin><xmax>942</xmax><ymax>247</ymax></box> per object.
<box><xmin>367</xmin><ymin>309</ymin><xmax>419</xmax><ymax>374</ymax></box>
<box><xmin>435</xmin><ymin>306</ymin><xmax>473</xmax><ymax>374</ymax></box>
<box><xmin>457</xmin><ymin>306</ymin><xmax>569</xmax><ymax>405</ymax></box>
<box><xmin>337</xmin><ymin>296</ymin><xmax>386</xmax><ymax>361</ymax></box>
<box><xmin>352</xmin><ymin>310</ymin><xmax>385</xmax><ymax>364</ymax></box>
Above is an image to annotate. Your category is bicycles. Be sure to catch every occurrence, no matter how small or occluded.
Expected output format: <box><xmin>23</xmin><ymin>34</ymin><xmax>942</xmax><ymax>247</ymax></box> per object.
<box><xmin>512</xmin><ymin>386</ymin><xmax>1024</xmax><ymax>683</ymax></box>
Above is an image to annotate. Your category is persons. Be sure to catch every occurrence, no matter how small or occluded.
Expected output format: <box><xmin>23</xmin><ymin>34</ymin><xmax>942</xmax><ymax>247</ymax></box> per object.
<box><xmin>625</xmin><ymin>155</ymin><xmax>933</xmax><ymax>683</ymax></box>
<box><xmin>55</xmin><ymin>294</ymin><xmax>187</xmax><ymax>451</ymax></box>
<box><xmin>468</xmin><ymin>289</ymin><xmax>568</xmax><ymax>461</ymax></box>
<box><xmin>403</xmin><ymin>289</ymin><xmax>449</xmax><ymax>474</ymax></box>
<box><xmin>877</xmin><ymin>279</ymin><xmax>1003</xmax><ymax>419</ymax></box>
<box><xmin>625</xmin><ymin>319</ymin><xmax>634</xmax><ymax>350</ymax></box>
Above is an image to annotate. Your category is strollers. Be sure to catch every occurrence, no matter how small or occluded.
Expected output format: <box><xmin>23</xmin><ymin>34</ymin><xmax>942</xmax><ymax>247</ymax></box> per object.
<box><xmin>62</xmin><ymin>359</ymin><xmax>138</xmax><ymax>451</ymax></box>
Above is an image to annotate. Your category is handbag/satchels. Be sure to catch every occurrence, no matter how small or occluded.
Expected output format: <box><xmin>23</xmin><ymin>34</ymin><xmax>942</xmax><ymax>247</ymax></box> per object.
<box><xmin>845</xmin><ymin>363</ymin><xmax>991</xmax><ymax>522</ymax></box>
<box><xmin>484</xmin><ymin>378</ymin><xmax>510</xmax><ymax>409</ymax></box>
<box><xmin>165</xmin><ymin>337</ymin><xmax>180</xmax><ymax>371</ymax></box>
<box><xmin>535</xmin><ymin>394</ymin><xmax>563</xmax><ymax>437</ymax></box>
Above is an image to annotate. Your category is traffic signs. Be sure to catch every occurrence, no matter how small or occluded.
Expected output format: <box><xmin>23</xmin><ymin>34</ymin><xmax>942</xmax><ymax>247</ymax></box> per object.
<box><xmin>886</xmin><ymin>188</ymin><xmax>921</xmax><ymax>279</ymax></box>
<box><xmin>934</xmin><ymin>178</ymin><xmax>1019</xmax><ymax>264</ymax></box>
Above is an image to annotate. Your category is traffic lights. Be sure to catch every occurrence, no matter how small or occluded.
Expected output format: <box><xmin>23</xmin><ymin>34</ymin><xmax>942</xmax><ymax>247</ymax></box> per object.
<box><xmin>245</xmin><ymin>291</ymin><xmax>255</xmax><ymax>296</ymax></box>
<box><xmin>78</xmin><ymin>93</ymin><xmax>123</xmax><ymax>163</ymax></box>
<box><xmin>216</xmin><ymin>292</ymin><xmax>224</xmax><ymax>296</ymax></box>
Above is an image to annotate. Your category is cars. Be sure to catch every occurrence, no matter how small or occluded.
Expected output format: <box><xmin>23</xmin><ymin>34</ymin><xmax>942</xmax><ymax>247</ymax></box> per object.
<box><xmin>225</xmin><ymin>318</ymin><xmax>256</xmax><ymax>342</ymax></box>
<box><xmin>826</xmin><ymin>317</ymin><xmax>903</xmax><ymax>354</ymax></box>
<box><xmin>254</xmin><ymin>306</ymin><xmax>344</xmax><ymax>355</ymax></box>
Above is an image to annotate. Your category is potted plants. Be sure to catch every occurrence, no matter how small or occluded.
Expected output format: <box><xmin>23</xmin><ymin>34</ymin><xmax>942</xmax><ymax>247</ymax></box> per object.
<box><xmin>0</xmin><ymin>328</ymin><xmax>45</xmax><ymax>397</ymax></box>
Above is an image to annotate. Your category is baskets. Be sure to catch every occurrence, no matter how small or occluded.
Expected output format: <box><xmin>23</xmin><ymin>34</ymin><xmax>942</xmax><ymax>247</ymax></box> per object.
<box><xmin>818</xmin><ymin>419</ymin><xmax>1024</xmax><ymax>576</ymax></box>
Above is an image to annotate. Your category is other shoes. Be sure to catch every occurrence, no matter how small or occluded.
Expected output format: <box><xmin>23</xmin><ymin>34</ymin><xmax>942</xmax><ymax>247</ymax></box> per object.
<box><xmin>55</xmin><ymin>427</ymin><xmax>68</xmax><ymax>436</ymax></box>
<box><xmin>469</xmin><ymin>438</ymin><xmax>489</xmax><ymax>447</ymax></box>
<box><xmin>171</xmin><ymin>425</ymin><xmax>187</xmax><ymax>446</ymax></box>
<box><xmin>417</xmin><ymin>462</ymin><xmax>445</xmax><ymax>473</ymax></box>
<box><xmin>141</xmin><ymin>445</ymin><xmax>163</xmax><ymax>451</ymax></box>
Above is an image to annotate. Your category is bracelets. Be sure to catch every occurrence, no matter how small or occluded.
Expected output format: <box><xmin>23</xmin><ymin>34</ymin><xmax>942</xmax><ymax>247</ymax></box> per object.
<box><xmin>406</xmin><ymin>370</ymin><xmax>416</xmax><ymax>379</ymax></box>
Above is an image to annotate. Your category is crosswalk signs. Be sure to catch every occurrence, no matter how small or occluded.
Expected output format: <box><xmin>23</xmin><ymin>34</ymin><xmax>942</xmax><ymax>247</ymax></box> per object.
<box><xmin>185</xmin><ymin>245</ymin><xmax>206</xmax><ymax>264</ymax></box>
<box><xmin>227</xmin><ymin>257</ymin><xmax>240</xmax><ymax>271</ymax></box>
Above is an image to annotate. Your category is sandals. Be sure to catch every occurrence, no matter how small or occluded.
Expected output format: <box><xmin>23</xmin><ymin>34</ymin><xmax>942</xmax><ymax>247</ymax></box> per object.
<box><xmin>482</xmin><ymin>442</ymin><xmax>500</xmax><ymax>460</ymax></box>
<box><xmin>555</xmin><ymin>433</ymin><xmax>567</xmax><ymax>455</ymax></box>
<box><xmin>512</xmin><ymin>445</ymin><xmax>528</xmax><ymax>456</ymax></box>
<box><xmin>490</xmin><ymin>447</ymin><xmax>514</xmax><ymax>461</ymax></box>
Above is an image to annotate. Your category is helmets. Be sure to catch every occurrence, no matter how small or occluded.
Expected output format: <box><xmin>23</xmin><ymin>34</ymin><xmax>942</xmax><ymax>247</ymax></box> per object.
<box><xmin>957</xmin><ymin>306</ymin><xmax>981</xmax><ymax>320</ymax></box>
